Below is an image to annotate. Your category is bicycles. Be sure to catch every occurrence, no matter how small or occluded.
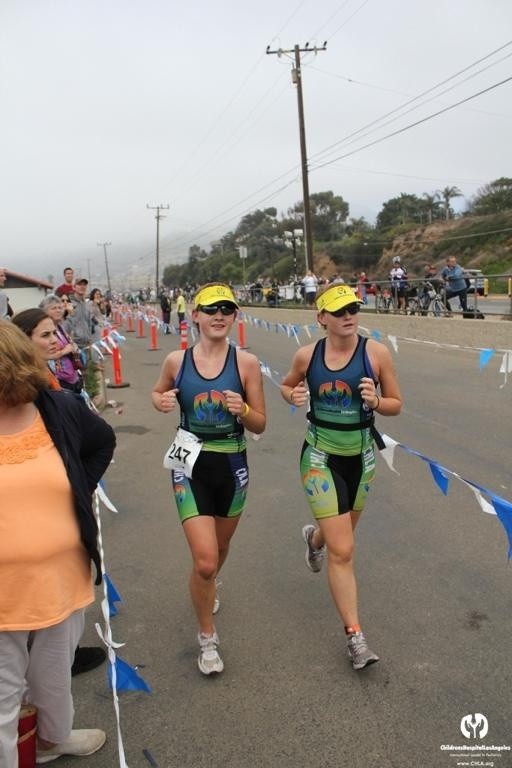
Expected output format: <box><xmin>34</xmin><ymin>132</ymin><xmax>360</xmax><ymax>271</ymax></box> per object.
<box><xmin>375</xmin><ymin>279</ymin><xmax>449</xmax><ymax>317</ymax></box>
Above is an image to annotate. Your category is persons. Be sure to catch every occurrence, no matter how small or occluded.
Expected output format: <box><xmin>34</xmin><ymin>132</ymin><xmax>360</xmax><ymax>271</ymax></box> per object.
<box><xmin>152</xmin><ymin>280</ymin><xmax>266</xmax><ymax>675</ymax></box>
<box><xmin>279</xmin><ymin>283</ymin><xmax>402</xmax><ymax>670</ymax></box>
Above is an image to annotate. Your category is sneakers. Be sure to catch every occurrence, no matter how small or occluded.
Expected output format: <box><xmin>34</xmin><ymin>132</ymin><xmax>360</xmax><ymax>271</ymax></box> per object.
<box><xmin>71</xmin><ymin>645</ymin><xmax>107</xmax><ymax>677</ymax></box>
<box><xmin>213</xmin><ymin>578</ymin><xmax>221</xmax><ymax>615</ymax></box>
<box><xmin>301</xmin><ymin>524</ymin><xmax>325</xmax><ymax>574</ymax></box>
<box><xmin>196</xmin><ymin>623</ymin><xmax>225</xmax><ymax>677</ymax></box>
<box><xmin>35</xmin><ymin>725</ymin><xmax>107</xmax><ymax>766</ymax></box>
<box><xmin>344</xmin><ymin>629</ymin><xmax>380</xmax><ymax>672</ymax></box>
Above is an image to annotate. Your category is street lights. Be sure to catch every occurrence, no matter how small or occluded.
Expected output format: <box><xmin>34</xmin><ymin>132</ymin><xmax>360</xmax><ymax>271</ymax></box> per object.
<box><xmin>231</xmin><ymin>231</ymin><xmax>251</xmax><ymax>290</ymax></box>
<box><xmin>284</xmin><ymin>227</ymin><xmax>304</xmax><ymax>288</ymax></box>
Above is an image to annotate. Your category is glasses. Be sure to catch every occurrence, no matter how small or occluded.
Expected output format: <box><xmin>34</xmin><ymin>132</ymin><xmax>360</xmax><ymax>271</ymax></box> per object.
<box><xmin>322</xmin><ymin>302</ymin><xmax>362</xmax><ymax>316</ymax></box>
<box><xmin>60</xmin><ymin>298</ymin><xmax>71</xmax><ymax>303</ymax></box>
<box><xmin>196</xmin><ymin>303</ymin><xmax>237</xmax><ymax>315</ymax></box>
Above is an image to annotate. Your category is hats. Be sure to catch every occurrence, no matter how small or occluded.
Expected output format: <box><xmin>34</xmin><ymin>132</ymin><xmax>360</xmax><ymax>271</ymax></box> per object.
<box><xmin>315</xmin><ymin>284</ymin><xmax>366</xmax><ymax>314</ymax></box>
<box><xmin>74</xmin><ymin>277</ymin><xmax>90</xmax><ymax>287</ymax></box>
<box><xmin>193</xmin><ymin>284</ymin><xmax>241</xmax><ymax>310</ymax></box>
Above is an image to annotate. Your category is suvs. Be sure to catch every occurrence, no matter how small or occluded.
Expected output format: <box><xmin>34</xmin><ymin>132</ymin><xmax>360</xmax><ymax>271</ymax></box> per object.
<box><xmin>464</xmin><ymin>268</ymin><xmax>487</xmax><ymax>294</ymax></box>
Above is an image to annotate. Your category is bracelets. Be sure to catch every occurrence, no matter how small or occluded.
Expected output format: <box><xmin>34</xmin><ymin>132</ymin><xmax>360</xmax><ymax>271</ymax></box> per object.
<box><xmin>288</xmin><ymin>390</ymin><xmax>294</xmax><ymax>403</ymax></box>
<box><xmin>242</xmin><ymin>402</ymin><xmax>252</xmax><ymax>419</ymax></box>
<box><xmin>369</xmin><ymin>394</ymin><xmax>380</xmax><ymax>409</ymax></box>
